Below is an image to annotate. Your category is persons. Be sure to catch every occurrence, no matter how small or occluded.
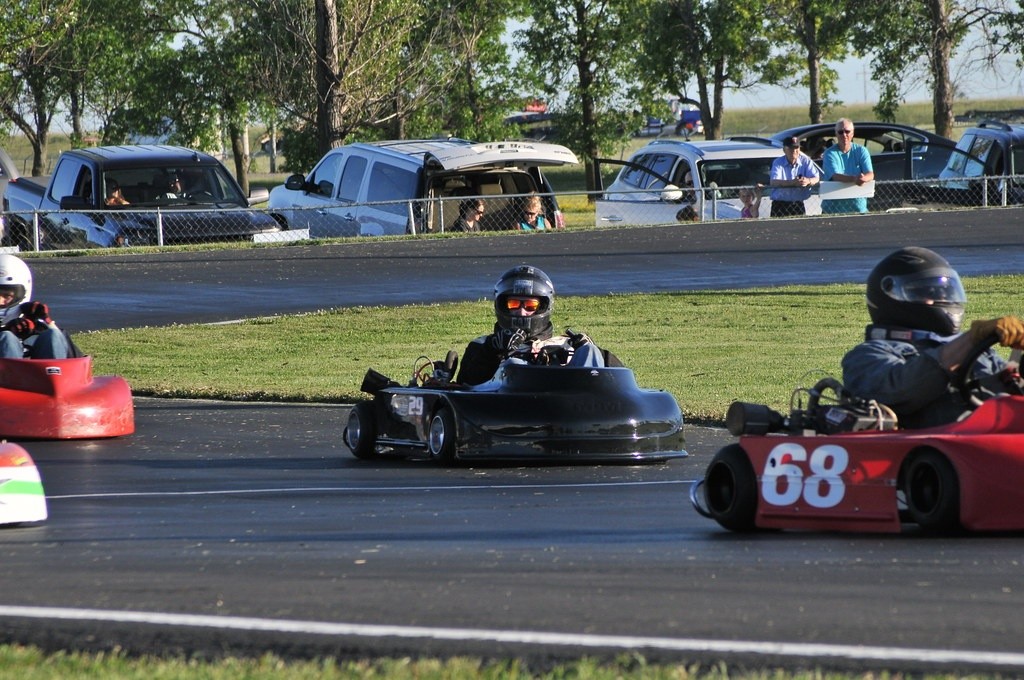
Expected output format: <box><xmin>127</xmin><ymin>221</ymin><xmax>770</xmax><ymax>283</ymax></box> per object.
<box><xmin>510</xmin><ymin>191</ymin><xmax>553</xmax><ymax>230</ymax></box>
<box><xmin>455</xmin><ymin>265</ymin><xmax>624</xmax><ymax>390</ymax></box>
<box><xmin>770</xmin><ymin>136</ymin><xmax>820</xmax><ymax>217</ymax></box>
<box><xmin>0</xmin><ymin>254</ymin><xmax>71</xmax><ymax>359</ymax></box>
<box><xmin>156</xmin><ymin>171</ymin><xmax>193</xmax><ymax>201</ymax></box>
<box><xmin>821</xmin><ymin>116</ymin><xmax>874</xmax><ymax>214</ymax></box>
<box><xmin>840</xmin><ymin>247</ymin><xmax>1024</xmax><ymax>426</ymax></box>
<box><xmin>106</xmin><ymin>177</ymin><xmax>131</xmax><ymax>206</ymax></box>
<box><xmin>452</xmin><ymin>198</ymin><xmax>484</xmax><ymax>233</ymax></box>
<box><xmin>675</xmin><ymin>205</ymin><xmax>698</xmax><ymax>221</ymax></box>
<box><xmin>738</xmin><ymin>183</ymin><xmax>764</xmax><ymax>218</ymax></box>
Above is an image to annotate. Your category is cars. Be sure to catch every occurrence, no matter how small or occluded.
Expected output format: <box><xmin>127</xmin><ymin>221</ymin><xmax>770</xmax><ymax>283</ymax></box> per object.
<box><xmin>266</xmin><ymin>138</ymin><xmax>580</xmax><ymax>239</ymax></box>
<box><xmin>767</xmin><ymin>122</ymin><xmax>957</xmax><ymax>212</ymax></box>
<box><xmin>631</xmin><ymin>97</ymin><xmax>704</xmax><ymax>136</ymax></box>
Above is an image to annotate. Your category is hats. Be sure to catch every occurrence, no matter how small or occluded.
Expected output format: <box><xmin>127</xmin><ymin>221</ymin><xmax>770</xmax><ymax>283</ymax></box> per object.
<box><xmin>784</xmin><ymin>137</ymin><xmax>799</xmax><ymax>147</ymax></box>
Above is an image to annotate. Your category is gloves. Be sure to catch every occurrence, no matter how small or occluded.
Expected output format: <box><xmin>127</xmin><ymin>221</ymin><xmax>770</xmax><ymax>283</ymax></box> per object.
<box><xmin>19</xmin><ymin>302</ymin><xmax>51</xmax><ymax>324</ymax></box>
<box><xmin>568</xmin><ymin>334</ymin><xmax>593</xmax><ymax>350</ymax></box>
<box><xmin>6</xmin><ymin>315</ymin><xmax>34</xmax><ymax>340</ymax></box>
<box><xmin>493</xmin><ymin>327</ymin><xmax>527</xmax><ymax>357</ymax></box>
<box><xmin>971</xmin><ymin>316</ymin><xmax>1024</xmax><ymax>349</ymax></box>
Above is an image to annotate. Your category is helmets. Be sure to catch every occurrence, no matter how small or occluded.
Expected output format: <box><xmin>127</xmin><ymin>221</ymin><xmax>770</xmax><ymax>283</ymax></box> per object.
<box><xmin>0</xmin><ymin>254</ymin><xmax>32</xmax><ymax>326</ymax></box>
<box><xmin>495</xmin><ymin>267</ymin><xmax>555</xmax><ymax>337</ymax></box>
<box><xmin>867</xmin><ymin>244</ymin><xmax>967</xmax><ymax>335</ymax></box>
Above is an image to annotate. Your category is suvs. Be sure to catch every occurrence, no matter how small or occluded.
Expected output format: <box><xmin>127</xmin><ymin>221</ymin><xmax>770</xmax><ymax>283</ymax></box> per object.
<box><xmin>593</xmin><ymin>135</ymin><xmax>825</xmax><ymax>229</ymax></box>
<box><xmin>903</xmin><ymin>119</ymin><xmax>1024</xmax><ymax>206</ymax></box>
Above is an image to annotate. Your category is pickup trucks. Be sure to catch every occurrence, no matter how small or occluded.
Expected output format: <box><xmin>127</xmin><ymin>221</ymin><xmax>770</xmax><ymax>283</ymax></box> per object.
<box><xmin>3</xmin><ymin>145</ymin><xmax>283</xmax><ymax>254</ymax></box>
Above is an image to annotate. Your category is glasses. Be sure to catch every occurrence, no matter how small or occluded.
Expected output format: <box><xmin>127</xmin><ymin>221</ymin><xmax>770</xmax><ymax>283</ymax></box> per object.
<box><xmin>523</xmin><ymin>210</ymin><xmax>539</xmax><ymax>216</ymax></box>
<box><xmin>472</xmin><ymin>207</ymin><xmax>483</xmax><ymax>215</ymax></box>
<box><xmin>837</xmin><ymin>130</ymin><xmax>854</xmax><ymax>135</ymax></box>
<box><xmin>507</xmin><ymin>298</ymin><xmax>546</xmax><ymax>313</ymax></box>
